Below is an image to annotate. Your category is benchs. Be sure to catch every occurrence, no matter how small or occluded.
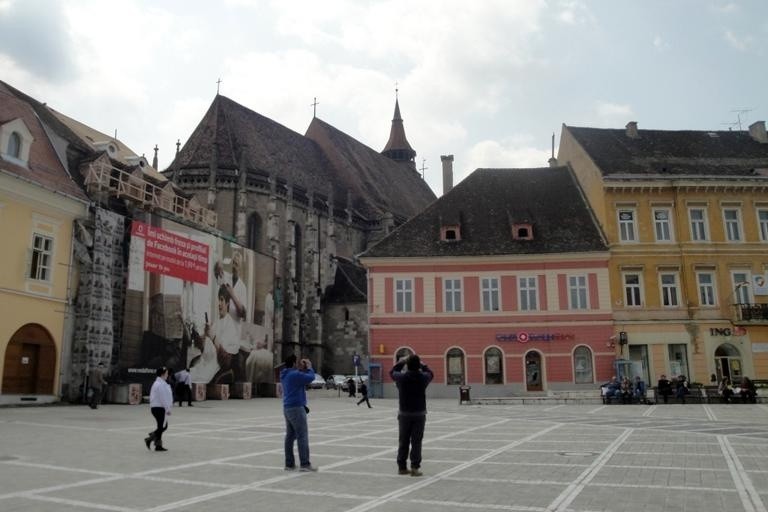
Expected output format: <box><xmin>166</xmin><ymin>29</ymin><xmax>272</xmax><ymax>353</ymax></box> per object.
<box><xmin>600</xmin><ymin>380</ymin><xmax>768</xmax><ymax>405</ymax></box>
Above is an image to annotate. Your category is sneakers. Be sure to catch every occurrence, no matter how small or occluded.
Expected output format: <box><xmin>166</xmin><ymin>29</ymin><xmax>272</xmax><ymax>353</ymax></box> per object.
<box><xmin>296</xmin><ymin>466</ymin><xmax>320</xmax><ymax>475</ymax></box>
<box><xmin>397</xmin><ymin>467</ymin><xmax>412</xmax><ymax>476</ymax></box>
<box><xmin>409</xmin><ymin>468</ymin><xmax>424</xmax><ymax>478</ymax></box>
<box><xmin>144</xmin><ymin>437</ymin><xmax>152</xmax><ymax>451</ymax></box>
<box><xmin>283</xmin><ymin>465</ymin><xmax>297</xmax><ymax>473</ymax></box>
<box><xmin>154</xmin><ymin>445</ymin><xmax>168</xmax><ymax>452</ymax></box>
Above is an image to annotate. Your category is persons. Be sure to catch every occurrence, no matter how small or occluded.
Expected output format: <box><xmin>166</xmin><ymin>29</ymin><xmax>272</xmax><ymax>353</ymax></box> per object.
<box><xmin>357</xmin><ymin>382</ymin><xmax>374</xmax><ymax>408</ymax></box>
<box><xmin>389</xmin><ymin>354</ymin><xmax>433</xmax><ymax>476</ymax></box>
<box><xmin>347</xmin><ymin>376</ymin><xmax>355</xmax><ymax>396</ymax></box>
<box><xmin>188</xmin><ymin>284</ymin><xmax>241</xmax><ymax>384</ymax></box>
<box><xmin>88</xmin><ymin>363</ymin><xmax>107</xmax><ymax>409</ymax></box>
<box><xmin>599</xmin><ymin>375</ymin><xmax>688</xmax><ymax>404</ymax></box>
<box><xmin>280</xmin><ymin>353</ymin><xmax>318</xmax><ymax>472</ymax></box>
<box><xmin>215</xmin><ymin>251</ymin><xmax>247</xmax><ymax>324</ymax></box>
<box><xmin>718</xmin><ymin>376</ymin><xmax>756</xmax><ymax>403</ymax></box>
<box><xmin>178</xmin><ymin>368</ymin><xmax>194</xmax><ymax>407</ymax></box>
<box><xmin>144</xmin><ymin>366</ymin><xmax>173</xmax><ymax>451</ymax></box>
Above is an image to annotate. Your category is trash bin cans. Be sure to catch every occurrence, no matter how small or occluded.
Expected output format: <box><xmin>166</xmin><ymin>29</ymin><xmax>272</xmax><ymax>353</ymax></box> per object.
<box><xmin>459</xmin><ymin>386</ymin><xmax>471</xmax><ymax>404</ymax></box>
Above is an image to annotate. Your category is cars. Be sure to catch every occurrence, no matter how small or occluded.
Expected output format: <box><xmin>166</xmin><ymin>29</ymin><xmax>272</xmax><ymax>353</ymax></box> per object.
<box><xmin>307</xmin><ymin>374</ymin><xmax>369</xmax><ymax>394</ymax></box>
